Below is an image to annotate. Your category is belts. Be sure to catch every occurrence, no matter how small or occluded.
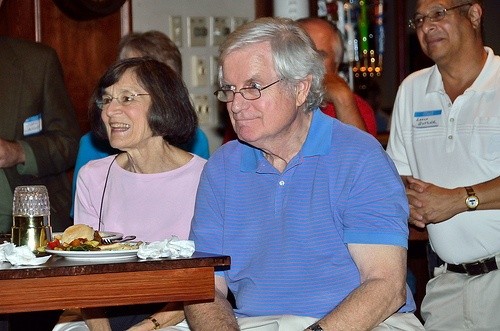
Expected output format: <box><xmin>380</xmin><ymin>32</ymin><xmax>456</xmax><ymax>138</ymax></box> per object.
<box><xmin>432</xmin><ymin>252</ymin><xmax>499</xmax><ymax>276</ymax></box>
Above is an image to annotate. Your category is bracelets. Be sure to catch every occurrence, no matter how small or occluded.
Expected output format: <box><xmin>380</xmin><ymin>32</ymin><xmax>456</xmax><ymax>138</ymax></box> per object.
<box><xmin>308</xmin><ymin>323</ymin><xmax>324</xmax><ymax>331</ymax></box>
<box><xmin>144</xmin><ymin>315</ymin><xmax>160</xmax><ymax>330</ymax></box>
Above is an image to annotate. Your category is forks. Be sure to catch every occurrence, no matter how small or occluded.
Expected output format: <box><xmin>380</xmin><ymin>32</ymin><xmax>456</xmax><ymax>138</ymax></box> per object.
<box><xmin>102</xmin><ymin>235</ymin><xmax>136</xmax><ymax>244</ymax></box>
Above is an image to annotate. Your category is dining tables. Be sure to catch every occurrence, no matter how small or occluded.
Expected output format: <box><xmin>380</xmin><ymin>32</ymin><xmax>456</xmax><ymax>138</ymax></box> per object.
<box><xmin>0</xmin><ymin>233</ymin><xmax>231</xmax><ymax>315</ymax></box>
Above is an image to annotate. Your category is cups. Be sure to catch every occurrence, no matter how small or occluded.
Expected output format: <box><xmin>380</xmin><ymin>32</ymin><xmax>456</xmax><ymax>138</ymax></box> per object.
<box><xmin>11</xmin><ymin>185</ymin><xmax>53</xmax><ymax>254</ymax></box>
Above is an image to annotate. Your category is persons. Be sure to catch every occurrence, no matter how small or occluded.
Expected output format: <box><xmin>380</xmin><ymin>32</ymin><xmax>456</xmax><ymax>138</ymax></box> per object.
<box><xmin>52</xmin><ymin>56</ymin><xmax>208</xmax><ymax>331</ymax></box>
<box><xmin>222</xmin><ymin>16</ymin><xmax>378</xmax><ymax>145</ymax></box>
<box><xmin>0</xmin><ymin>34</ymin><xmax>81</xmax><ymax>238</ymax></box>
<box><xmin>182</xmin><ymin>16</ymin><xmax>426</xmax><ymax>331</ymax></box>
<box><xmin>385</xmin><ymin>0</ymin><xmax>500</xmax><ymax>331</ymax></box>
<box><xmin>70</xmin><ymin>30</ymin><xmax>211</xmax><ymax>219</ymax></box>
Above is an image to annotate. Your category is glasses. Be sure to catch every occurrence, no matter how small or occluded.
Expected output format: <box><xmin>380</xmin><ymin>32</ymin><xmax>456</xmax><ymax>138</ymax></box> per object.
<box><xmin>95</xmin><ymin>92</ymin><xmax>152</xmax><ymax>110</ymax></box>
<box><xmin>408</xmin><ymin>3</ymin><xmax>474</xmax><ymax>31</ymax></box>
<box><xmin>212</xmin><ymin>78</ymin><xmax>287</xmax><ymax>103</ymax></box>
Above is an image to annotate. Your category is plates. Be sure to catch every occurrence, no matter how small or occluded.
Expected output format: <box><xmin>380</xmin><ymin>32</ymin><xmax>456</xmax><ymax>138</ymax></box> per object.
<box><xmin>46</xmin><ymin>250</ymin><xmax>138</xmax><ymax>260</ymax></box>
<box><xmin>54</xmin><ymin>231</ymin><xmax>123</xmax><ymax>240</ymax></box>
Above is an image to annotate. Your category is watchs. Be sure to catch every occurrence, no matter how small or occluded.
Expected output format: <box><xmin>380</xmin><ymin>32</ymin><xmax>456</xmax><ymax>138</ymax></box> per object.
<box><xmin>464</xmin><ymin>186</ymin><xmax>480</xmax><ymax>212</ymax></box>
